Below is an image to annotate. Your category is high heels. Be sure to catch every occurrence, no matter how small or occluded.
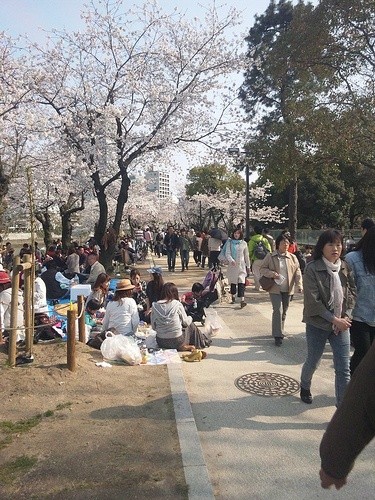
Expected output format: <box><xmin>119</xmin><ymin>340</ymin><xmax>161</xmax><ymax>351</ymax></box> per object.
<box><xmin>184</xmin><ymin>351</ymin><xmax>202</xmax><ymax>362</ymax></box>
<box><xmin>182</xmin><ymin>349</ymin><xmax>199</xmax><ymax>358</ymax></box>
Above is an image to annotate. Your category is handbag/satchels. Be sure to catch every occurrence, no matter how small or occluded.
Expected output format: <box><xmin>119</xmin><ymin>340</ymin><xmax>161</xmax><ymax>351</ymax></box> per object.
<box><xmin>258</xmin><ymin>253</ymin><xmax>276</xmax><ymax>292</ymax></box>
<box><xmin>217</xmin><ymin>238</ymin><xmax>231</xmax><ymax>265</ymax></box>
<box><xmin>100</xmin><ymin>331</ymin><xmax>142</xmax><ymax>366</ymax></box>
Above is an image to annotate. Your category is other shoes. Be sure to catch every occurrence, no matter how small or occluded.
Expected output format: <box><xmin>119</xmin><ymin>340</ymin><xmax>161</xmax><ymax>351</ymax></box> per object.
<box><xmin>241</xmin><ymin>301</ymin><xmax>247</xmax><ymax>308</ymax></box>
<box><xmin>230</xmin><ymin>297</ymin><xmax>235</xmax><ymax>304</ymax></box>
<box><xmin>275</xmin><ymin>337</ymin><xmax>283</xmax><ymax>346</ymax></box>
<box><xmin>301</xmin><ymin>389</ymin><xmax>312</xmax><ymax>403</ymax></box>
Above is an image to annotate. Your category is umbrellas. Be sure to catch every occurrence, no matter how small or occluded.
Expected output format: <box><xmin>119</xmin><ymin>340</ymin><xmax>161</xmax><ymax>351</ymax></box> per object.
<box><xmin>209</xmin><ymin>229</ymin><xmax>228</xmax><ymax>240</ymax></box>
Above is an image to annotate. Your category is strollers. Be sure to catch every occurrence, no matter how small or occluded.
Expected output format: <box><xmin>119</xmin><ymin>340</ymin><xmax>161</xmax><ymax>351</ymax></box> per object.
<box><xmin>181</xmin><ymin>267</ymin><xmax>222</xmax><ymax>327</ymax></box>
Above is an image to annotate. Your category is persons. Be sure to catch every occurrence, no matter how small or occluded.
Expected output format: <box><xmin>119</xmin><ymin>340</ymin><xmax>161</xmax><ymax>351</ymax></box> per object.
<box><xmin>319</xmin><ymin>341</ymin><xmax>375</xmax><ymax>490</ymax></box>
<box><xmin>237</xmin><ymin>219</ymin><xmax>245</xmax><ymax>233</ymax></box>
<box><xmin>300</xmin><ymin>218</ymin><xmax>375</xmax><ymax>410</ymax></box>
<box><xmin>140</xmin><ymin>273</ymin><xmax>164</xmax><ymax>323</ymax></box>
<box><xmin>101</xmin><ymin>279</ymin><xmax>139</xmax><ymax>337</ymax></box>
<box><xmin>0</xmin><ymin>223</ymin><xmax>112</xmax><ymax>344</ymax></box>
<box><xmin>259</xmin><ymin>234</ymin><xmax>304</xmax><ymax>344</ymax></box>
<box><xmin>114</xmin><ymin>222</ymin><xmax>230</xmax><ymax>272</ymax></box>
<box><xmin>248</xmin><ymin>225</ymin><xmax>313</xmax><ymax>301</ymax></box>
<box><xmin>151</xmin><ymin>282</ymin><xmax>212</xmax><ymax>350</ymax></box>
<box><xmin>129</xmin><ymin>269</ymin><xmax>148</xmax><ymax>312</ymax></box>
<box><xmin>181</xmin><ymin>283</ymin><xmax>204</xmax><ymax>306</ymax></box>
<box><xmin>226</xmin><ymin>228</ymin><xmax>250</xmax><ymax>307</ymax></box>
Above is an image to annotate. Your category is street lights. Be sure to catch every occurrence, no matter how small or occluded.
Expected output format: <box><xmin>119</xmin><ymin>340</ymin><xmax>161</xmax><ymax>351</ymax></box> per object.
<box><xmin>227</xmin><ymin>147</ymin><xmax>262</xmax><ymax>243</ymax></box>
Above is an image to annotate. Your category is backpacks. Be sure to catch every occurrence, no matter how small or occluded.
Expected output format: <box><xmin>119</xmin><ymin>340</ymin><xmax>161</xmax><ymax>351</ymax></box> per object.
<box><xmin>252</xmin><ymin>241</ymin><xmax>267</xmax><ymax>258</ymax></box>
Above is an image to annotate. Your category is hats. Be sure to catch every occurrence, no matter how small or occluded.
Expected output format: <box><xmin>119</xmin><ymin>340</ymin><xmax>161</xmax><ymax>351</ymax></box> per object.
<box><xmin>115</xmin><ymin>279</ymin><xmax>136</xmax><ymax>290</ymax></box>
<box><xmin>147</xmin><ymin>266</ymin><xmax>161</xmax><ymax>274</ymax></box>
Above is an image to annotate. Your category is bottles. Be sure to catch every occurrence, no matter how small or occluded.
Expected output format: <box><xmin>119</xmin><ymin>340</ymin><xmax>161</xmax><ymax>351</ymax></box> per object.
<box><xmin>193</xmin><ymin>299</ymin><xmax>197</xmax><ymax>308</ymax></box>
<box><xmin>140</xmin><ymin>344</ymin><xmax>148</xmax><ymax>364</ymax></box>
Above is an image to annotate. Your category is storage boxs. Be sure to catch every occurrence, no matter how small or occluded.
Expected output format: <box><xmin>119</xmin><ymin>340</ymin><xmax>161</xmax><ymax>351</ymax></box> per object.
<box><xmin>70</xmin><ymin>284</ymin><xmax>91</xmax><ymax>301</ymax></box>
